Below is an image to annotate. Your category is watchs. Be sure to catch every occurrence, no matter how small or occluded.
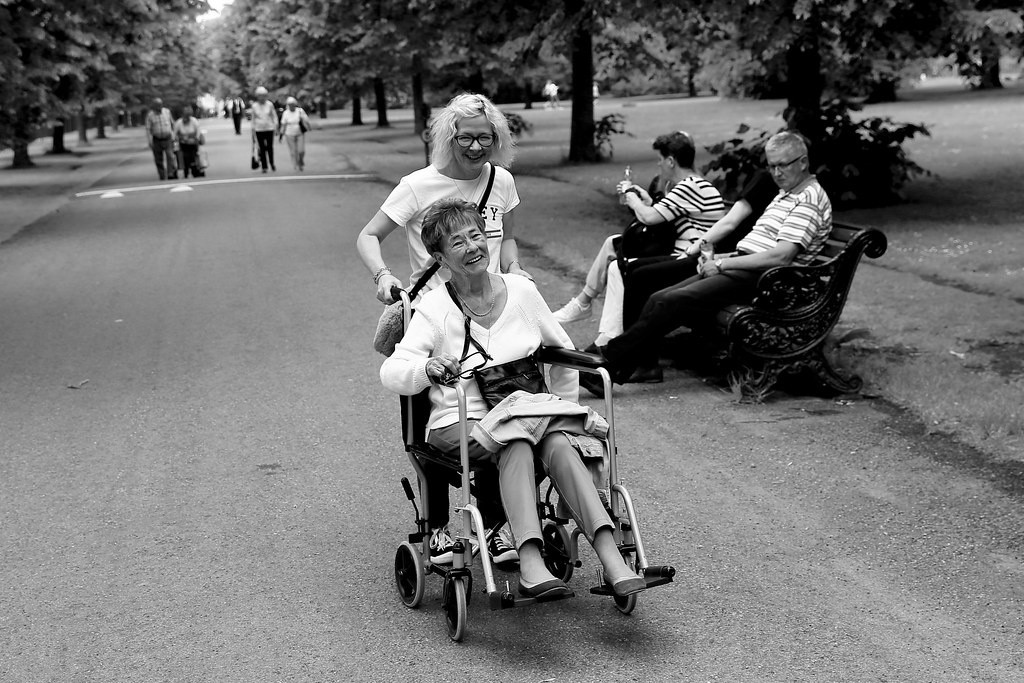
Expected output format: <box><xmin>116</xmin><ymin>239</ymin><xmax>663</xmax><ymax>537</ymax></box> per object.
<box><xmin>715</xmin><ymin>257</ymin><xmax>722</xmax><ymax>272</ymax></box>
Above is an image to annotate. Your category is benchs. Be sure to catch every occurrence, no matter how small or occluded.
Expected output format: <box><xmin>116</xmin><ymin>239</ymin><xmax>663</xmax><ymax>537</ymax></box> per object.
<box><xmin>683</xmin><ymin>200</ymin><xmax>887</xmax><ymax>398</ymax></box>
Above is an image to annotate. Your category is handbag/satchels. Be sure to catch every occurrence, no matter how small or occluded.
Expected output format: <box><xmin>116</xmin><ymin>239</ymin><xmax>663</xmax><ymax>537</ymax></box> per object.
<box><xmin>252</xmin><ymin>156</ymin><xmax>260</xmax><ymax>169</ymax></box>
<box><xmin>299</xmin><ymin>109</ymin><xmax>308</xmax><ymax>134</ymax></box>
<box><xmin>476</xmin><ymin>356</ymin><xmax>549</xmax><ymax>411</ymax></box>
<box><xmin>196</xmin><ymin>149</ymin><xmax>208</xmax><ymax>171</ymax></box>
<box><xmin>372</xmin><ymin>299</ymin><xmax>406</xmax><ymax>358</ymax></box>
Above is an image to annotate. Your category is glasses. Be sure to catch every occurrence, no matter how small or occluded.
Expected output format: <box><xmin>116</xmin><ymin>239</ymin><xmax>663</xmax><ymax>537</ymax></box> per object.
<box><xmin>454</xmin><ymin>133</ymin><xmax>496</xmax><ymax>147</ymax></box>
<box><xmin>767</xmin><ymin>154</ymin><xmax>802</xmax><ymax>171</ymax></box>
<box><xmin>441</xmin><ymin>352</ymin><xmax>493</xmax><ymax>384</ymax></box>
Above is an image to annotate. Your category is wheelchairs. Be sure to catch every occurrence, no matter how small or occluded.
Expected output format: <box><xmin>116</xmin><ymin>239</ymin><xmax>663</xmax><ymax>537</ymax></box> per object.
<box><xmin>389</xmin><ymin>284</ymin><xmax>677</xmax><ymax>642</ymax></box>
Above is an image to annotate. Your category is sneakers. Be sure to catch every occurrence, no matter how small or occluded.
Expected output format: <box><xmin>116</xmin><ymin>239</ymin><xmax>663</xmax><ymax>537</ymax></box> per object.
<box><xmin>576</xmin><ymin>343</ymin><xmax>613</xmax><ymax>398</ymax></box>
<box><xmin>552</xmin><ymin>297</ymin><xmax>592</xmax><ymax>322</ymax></box>
<box><xmin>628</xmin><ymin>366</ymin><xmax>663</xmax><ymax>384</ymax></box>
<box><xmin>488</xmin><ymin>528</ymin><xmax>520</xmax><ymax>563</ymax></box>
<box><xmin>430</xmin><ymin>528</ymin><xmax>454</xmax><ymax>564</ymax></box>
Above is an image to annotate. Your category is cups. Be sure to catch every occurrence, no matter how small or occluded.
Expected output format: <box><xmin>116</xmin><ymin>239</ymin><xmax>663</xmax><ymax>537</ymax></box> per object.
<box><xmin>701</xmin><ymin>242</ymin><xmax>714</xmax><ymax>264</ymax></box>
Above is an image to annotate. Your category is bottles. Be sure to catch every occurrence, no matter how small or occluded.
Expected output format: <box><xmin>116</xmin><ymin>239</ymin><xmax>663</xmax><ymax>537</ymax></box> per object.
<box><xmin>618</xmin><ymin>165</ymin><xmax>633</xmax><ymax>204</ymax></box>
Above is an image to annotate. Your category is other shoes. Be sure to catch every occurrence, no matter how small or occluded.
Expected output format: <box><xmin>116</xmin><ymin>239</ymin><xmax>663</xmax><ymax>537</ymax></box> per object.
<box><xmin>519</xmin><ymin>579</ymin><xmax>569</xmax><ymax>598</ymax></box>
<box><xmin>603</xmin><ymin>571</ymin><xmax>647</xmax><ymax>596</ymax></box>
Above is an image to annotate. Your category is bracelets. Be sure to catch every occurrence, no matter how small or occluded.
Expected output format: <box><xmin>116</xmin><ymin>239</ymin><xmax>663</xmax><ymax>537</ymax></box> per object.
<box><xmin>507</xmin><ymin>261</ymin><xmax>523</xmax><ymax>273</ymax></box>
<box><xmin>685</xmin><ymin>247</ymin><xmax>690</xmax><ymax>256</ymax></box>
<box><xmin>373</xmin><ymin>268</ymin><xmax>392</xmax><ymax>285</ymax></box>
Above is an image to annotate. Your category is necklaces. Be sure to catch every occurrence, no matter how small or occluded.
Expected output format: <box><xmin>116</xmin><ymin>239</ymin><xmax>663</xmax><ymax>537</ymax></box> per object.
<box><xmin>447</xmin><ymin>165</ymin><xmax>482</xmax><ymax>202</ymax></box>
<box><xmin>453</xmin><ymin>289</ymin><xmax>492</xmax><ymax>356</ymax></box>
<box><xmin>450</xmin><ymin>276</ymin><xmax>495</xmax><ymax>316</ymax></box>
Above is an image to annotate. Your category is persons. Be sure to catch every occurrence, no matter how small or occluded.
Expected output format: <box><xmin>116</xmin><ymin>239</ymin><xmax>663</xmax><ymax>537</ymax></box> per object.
<box><xmin>278</xmin><ymin>96</ymin><xmax>311</xmax><ymax>171</ymax></box>
<box><xmin>380</xmin><ymin>196</ymin><xmax>645</xmax><ymax>596</ymax></box>
<box><xmin>544</xmin><ymin>80</ymin><xmax>560</xmax><ymax>107</ymax></box>
<box><xmin>251</xmin><ymin>87</ymin><xmax>279</xmax><ymax>172</ymax></box>
<box><xmin>623</xmin><ymin>131</ymin><xmax>811</xmax><ymax>383</ymax></box>
<box><xmin>576</xmin><ymin>131</ymin><xmax>833</xmax><ymax>396</ymax></box>
<box><xmin>146</xmin><ymin>98</ymin><xmax>176</xmax><ymax>180</ymax></box>
<box><xmin>172</xmin><ymin>106</ymin><xmax>201</xmax><ymax>178</ymax></box>
<box><xmin>553</xmin><ymin>131</ymin><xmax>726</xmax><ymax>354</ymax></box>
<box><xmin>593</xmin><ymin>82</ymin><xmax>599</xmax><ymax>105</ymax></box>
<box><xmin>358</xmin><ymin>95</ymin><xmax>535</xmax><ymax>563</ymax></box>
<box><xmin>227</xmin><ymin>94</ymin><xmax>245</xmax><ymax>135</ymax></box>
